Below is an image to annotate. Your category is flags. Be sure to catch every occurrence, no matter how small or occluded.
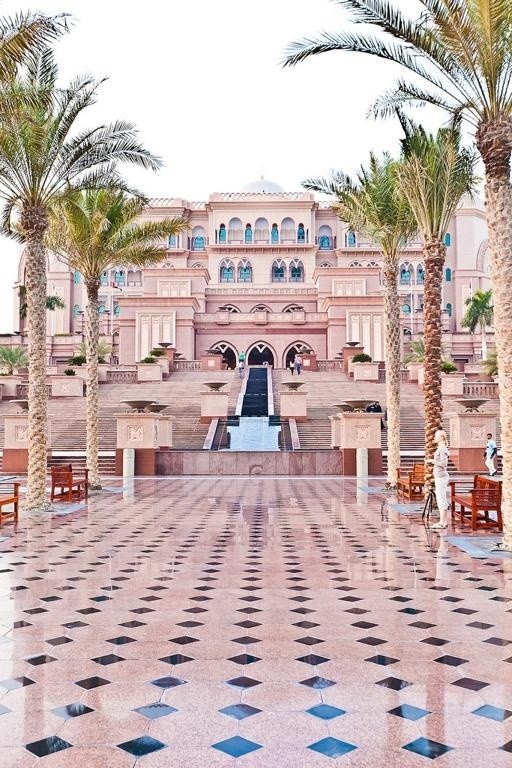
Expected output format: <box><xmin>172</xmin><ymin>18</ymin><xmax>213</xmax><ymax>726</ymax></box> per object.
<box><xmin>112</xmin><ymin>280</ymin><xmax>123</xmax><ymax>291</ymax></box>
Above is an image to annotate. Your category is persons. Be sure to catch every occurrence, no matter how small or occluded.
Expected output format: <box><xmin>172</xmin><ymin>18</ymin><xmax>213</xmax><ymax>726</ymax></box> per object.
<box><xmin>425</xmin><ymin>430</ymin><xmax>451</xmax><ymax>529</ymax></box>
<box><xmin>294</xmin><ymin>354</ymin><xmax>303</xmax><ymax>375</ymax></box>
<box><xmin>483</xmin><ymin>433</ymin><xmax>497</xmax><ymax>477</ymax></box>
<box><xmin>239</xmin><ymin>351</ymin><xmax>246</xmax><ymax>362</ymax></box>
<box><xmin>237</xmin><ymin>360</ymin><xmax>245</xmax><ymax>379</ymax></box>
<box><xmin>289</xmin><ymin>356</ymin><xmax>295</xmax><ymax>376</ymax></box>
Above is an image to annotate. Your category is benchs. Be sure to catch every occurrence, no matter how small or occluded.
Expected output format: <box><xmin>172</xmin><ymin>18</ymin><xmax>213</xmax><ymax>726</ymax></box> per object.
<box><xmin>50</xmin><ymin>463</ymin><xmax>90</xmax><ymax>504</ymax></box>
<box><xmin>0</xmin><ymin>482</ymin><xmax>21</xmax><ymax>526</ymax></box>
<box><xmin>395</xmin><ymin>462</ymin><xmax>431</xmax><ymax>502</ymax></box>
<box><xmin>447</xmin><ymin>473</ymin><xmax>502</xmax><ymax>533</ymax></box>
